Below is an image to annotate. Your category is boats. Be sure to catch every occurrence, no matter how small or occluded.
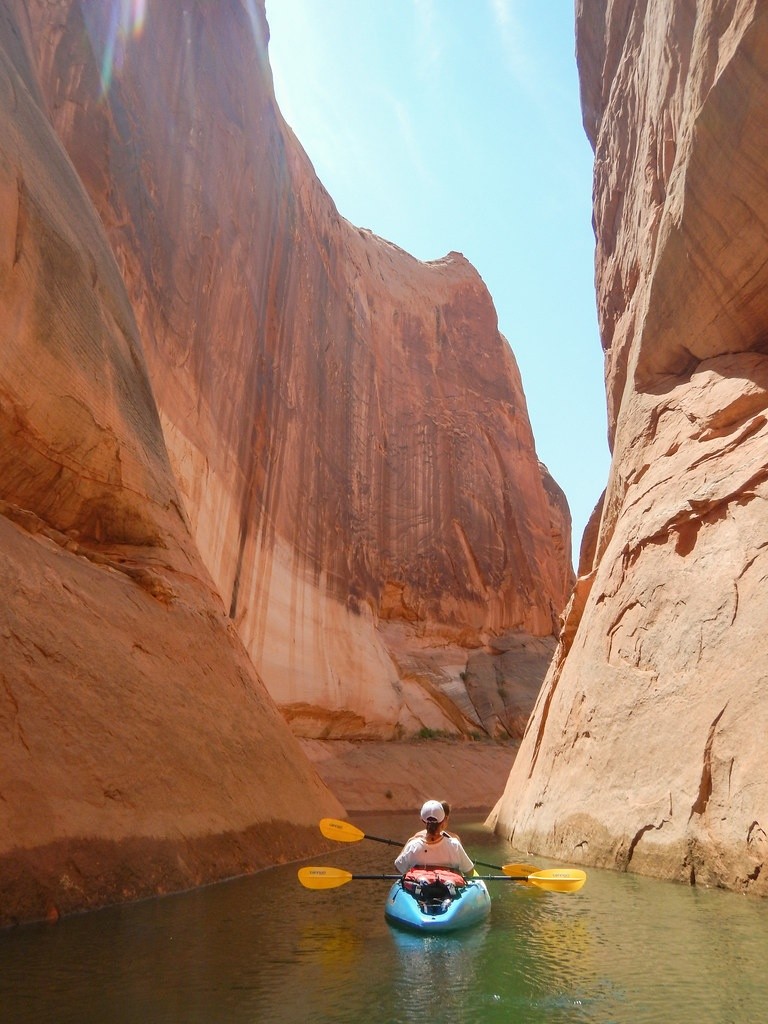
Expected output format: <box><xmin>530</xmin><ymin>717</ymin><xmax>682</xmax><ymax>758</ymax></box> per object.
<box><xmin>383</xmin><ymin>868</ymin><xmax>492</xmax><ymax>936</ymax></box>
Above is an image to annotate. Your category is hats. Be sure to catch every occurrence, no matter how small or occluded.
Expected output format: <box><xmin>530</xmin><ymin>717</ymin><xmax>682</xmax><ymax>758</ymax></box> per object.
<box><xmin>421</xmin><ymin>800</ymin><xmax>445</xmax><ymax>824</ymax></box>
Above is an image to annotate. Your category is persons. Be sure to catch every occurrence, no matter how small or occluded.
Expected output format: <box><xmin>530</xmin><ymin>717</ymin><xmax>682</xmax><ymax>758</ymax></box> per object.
<box><xmin>394</xmin><ymin>800</ymin><xmax>475</xmax><ymax>880</ymax></box>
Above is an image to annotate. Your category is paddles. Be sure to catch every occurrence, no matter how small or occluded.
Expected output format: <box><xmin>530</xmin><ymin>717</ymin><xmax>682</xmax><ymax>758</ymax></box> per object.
<box><xmin>320</xmin><ymin>817</ymin><xmax>543</xmax><ymax>886</ymax></box>
<box><xmin>298</xmin><ymin>865</ymin><xmax>586</xmax><ymax>893</ymax></box>
